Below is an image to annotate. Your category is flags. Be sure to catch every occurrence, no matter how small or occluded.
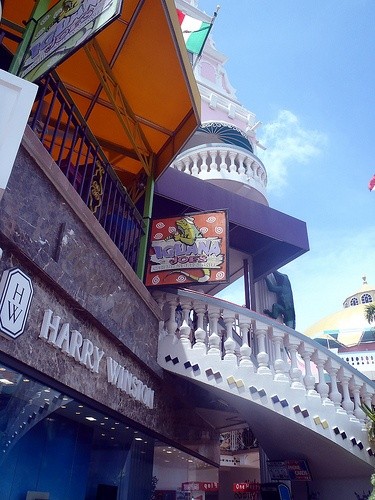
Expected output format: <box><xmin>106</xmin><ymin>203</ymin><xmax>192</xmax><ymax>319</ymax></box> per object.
<box><xmin>175</xmin><ymin>0</ymin><xmax>215</xmax><ymax>54</ymax></box>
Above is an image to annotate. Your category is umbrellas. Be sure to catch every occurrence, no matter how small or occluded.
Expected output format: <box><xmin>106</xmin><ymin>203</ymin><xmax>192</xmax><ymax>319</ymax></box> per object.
<box><xmin>313</xmin><ymin>333</ymin><xmax>348</xmax><ymax>353</ymax></box>
